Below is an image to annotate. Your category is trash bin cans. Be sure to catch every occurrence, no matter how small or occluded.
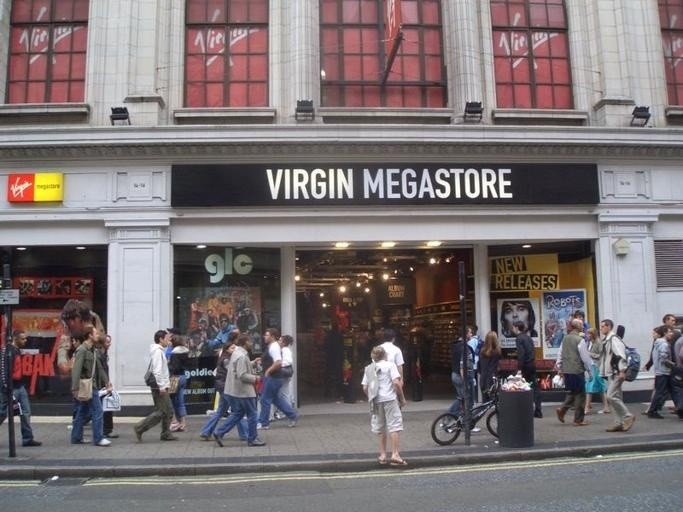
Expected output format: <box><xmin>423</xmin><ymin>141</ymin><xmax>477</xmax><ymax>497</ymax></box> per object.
<box><xmin>499</xmin><ymin>388</ymin><xmax>534</xmax><ymax>448</ymax></box>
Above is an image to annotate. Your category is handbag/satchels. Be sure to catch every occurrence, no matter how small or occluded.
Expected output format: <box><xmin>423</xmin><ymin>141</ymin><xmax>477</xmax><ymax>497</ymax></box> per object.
<box><xmin>144</xmin><ymin>370</ymin><xmax>157</xmax><ymax>388</ymax></box>
<box><xmin>623</xmin><ymin>348</ymin><xmax>641</xmax><ymax>381</ymax></box>
<box><xmin>75</xmin><ymin>379</ymin><xmax>93</xmax><ymax>401</ymax></box>
<box><xmin>167</xmin><ymin>377</ymin><xmax>180</xmax><ymax>394</ymax></box>
<box><xmin>273</xmin><ymin>366</ymin><xmax>294</xmax><ymax>378</ymax></box>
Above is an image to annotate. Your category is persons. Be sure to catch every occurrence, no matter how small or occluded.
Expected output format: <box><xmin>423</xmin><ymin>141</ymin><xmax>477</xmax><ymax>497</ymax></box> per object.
<box><xmin>512</xmin><ymin>320</ymin><xmax>543</xmax><ymax>418</ymax></box>
<box><xmin>133</xmin><ymin>327</ymin><xmax>190</xmax><ymax>445</ymax></box>
<box><xmin>556</xmin><ymin>313</ymin><xmax>683</xmax><ymax>432</ymax></box>
<box><xmin>56</xmin><ymin>298</ymin><xmax>105</xmax><ymax>373</ymax></box>
<box><xmin>0</xmin><ymin>328</ymin><xmax>42</xmax><ymax>447</ymax></box>
<box><xmin>188</xmin><ymin>292</ymin><xmax>261</xmax><ymax>354</ymax></box>
<box><xmin>441</xmin><ymin>323</ymin><xmax>502</xmax><ymax>432</ymax></box>
<box><xmin>199</xmin><ymin>327</ymin><xmax>302</xmax><ymax>447</ymax></box>
<box><xmin>500</xmin><ymin>301</ymin><xmax>541</xmax><ymax>340</ymax></box>
<box><xmin>62</xmin><ymin>327</ymin><xmax>121</xmax><ymax>448</ymax></box>
<box><xmin>323</xmin><ymin>320</ymin><xmax>434</xmax><ymax>466</ymax></box>
<box><xmin>544</xmin><ymin>308</ymin><xmax>589</xmax><ymax>346</ymax></box>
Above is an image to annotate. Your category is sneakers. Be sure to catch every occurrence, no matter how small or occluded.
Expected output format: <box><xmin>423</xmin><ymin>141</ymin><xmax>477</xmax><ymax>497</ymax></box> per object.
<box><xmin>256</xmin><ymin>421</ymin><xmax>269</xmax><ymax>431</ymax></box>
<box><xmin>641</xmin><ymin>406</ymin><xmax>683</xmax><ymax>420</ymax></box>
<box><xmin>248</xmin><ymin>437</ymin><xmax>265</xmax><ymax>446</ymax></box>
<box><xmin>134</xmin><ymin>426</ymin><xmax>144</xmax><ymax>443</ymax></box>
<box><xmin>471</xmin><ymin>427</ymin><xmax>481</xmax><ymax>431</ymax></box>
<box><xmin>557</xmin><ymin>407</ymin><xmax>565</xmax><ymax>423</ymax></box>
<box><xmin>534</xmin><ymin>412</ymin><xmax>542</xmax><ymax>417</ymax></box>
<box><xmin>607</xmin><ymin>416</ymin><xmax>635</xmax><ymax>431</ymax></box>
<box><xmin>160</xmin><ymin>418</ymin><xmax>185</xmax><ymax>441</ymax></box>
<box><xmin>196</xmin><ymin>429</ymin><xmax>225</xmax><ymax>447</ymax></box>
<box><xmin>597</xmin><ymin>409</ymin><xmax>611</xmax><ymax>414</ymax></box>
<box><xmin>67</xmin><ymin>425</ymin><xmax>119</xmax><ymax>445</ymax></box>
<box><xmin>574</xmin><ymin>419</ymin><xmax>587</xmax><ymax>426</ymax></box>
<box><xmin>289</xmin><ymin>414</ymin><xmax>299</xmax><ymax>426</ymax></box>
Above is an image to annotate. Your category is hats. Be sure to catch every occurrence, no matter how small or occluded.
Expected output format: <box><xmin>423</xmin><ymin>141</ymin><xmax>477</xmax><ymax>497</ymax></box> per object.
<box><xmin>167</xmin><ymin>328</ymin><xmax>180</xmax><ymax>335</ymax></box>
<box><xmin>219</xmin><ymin>314</ymin><xmax>229</xmax><ymax>321</ymax></box>
<box><xmin>199</xmin><ymin>317</ymin><xmax>206</xmax><ymax>322</ymax></box>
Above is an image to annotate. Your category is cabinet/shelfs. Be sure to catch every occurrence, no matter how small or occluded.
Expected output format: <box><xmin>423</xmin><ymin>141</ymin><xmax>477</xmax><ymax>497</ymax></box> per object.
<box><xmin>297</xmin><ymin>299</ymin><xmax>473</xmax><ymax>387</ymax></box>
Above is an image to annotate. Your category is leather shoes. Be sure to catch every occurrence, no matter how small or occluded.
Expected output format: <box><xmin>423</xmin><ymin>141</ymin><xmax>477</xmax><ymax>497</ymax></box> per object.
<box><xmin>24</xmin><ymin>439</ymin><xmax>41</xmax><ymax>446</ymax></box>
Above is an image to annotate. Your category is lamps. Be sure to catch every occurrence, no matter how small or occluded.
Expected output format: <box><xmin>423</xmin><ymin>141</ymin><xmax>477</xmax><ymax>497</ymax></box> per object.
<box><xmin>109</xmin><ymin>107</ymin><xmax>132</xmax><ymax>125</ymax></box>
<box><xmin>295</xmin><ymin>100</ymin><xmax>315</xmax><ymax>120</ymax></box>
<box><xmin>630</xmin><ymin>106</ymin><xmax>651</xmax><ymax>127</ymax></box>
<box><xmin>464</xmin><ymin>102</ymin><xmax>484</xmax><ymax>122</ymax></box>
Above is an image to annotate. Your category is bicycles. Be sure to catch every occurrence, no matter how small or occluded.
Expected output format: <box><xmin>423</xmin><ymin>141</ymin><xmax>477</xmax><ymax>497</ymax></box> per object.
<box><xmin>430</xmin><ymin>377</ymin><xmax>499</xmax><ymax>446</ymax></box>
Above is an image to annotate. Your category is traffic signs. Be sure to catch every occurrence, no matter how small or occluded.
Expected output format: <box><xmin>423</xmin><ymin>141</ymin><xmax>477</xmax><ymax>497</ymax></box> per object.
<box><xmin>0</xmin><ymin>289</ymin><xmax>19</xmax><ymax>304</ymax></box>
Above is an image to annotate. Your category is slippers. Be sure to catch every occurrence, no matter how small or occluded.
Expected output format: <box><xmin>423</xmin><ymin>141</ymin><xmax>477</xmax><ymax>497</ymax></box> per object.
<box><xmin>377</xmin><ymin>455</ymin><xmax>407</xmax><ymax>466</ymax></box>
